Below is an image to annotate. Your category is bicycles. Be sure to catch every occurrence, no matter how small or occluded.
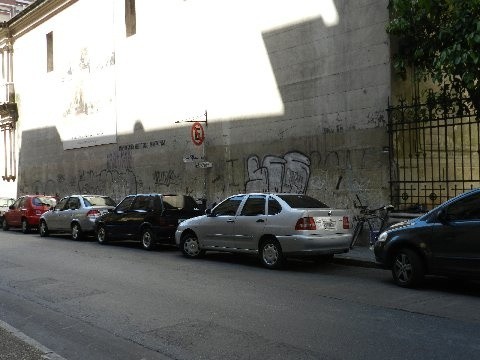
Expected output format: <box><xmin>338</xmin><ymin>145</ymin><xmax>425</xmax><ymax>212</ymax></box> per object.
<box><xmin>350</xmin><ymin>194</ymin><xmax>399</xmax><ymax>249</ymax></box>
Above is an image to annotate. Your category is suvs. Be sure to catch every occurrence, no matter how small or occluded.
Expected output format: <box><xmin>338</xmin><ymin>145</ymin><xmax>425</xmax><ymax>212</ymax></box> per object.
<box><xmin>94</xmin><ymin>192</ymin><xmax>201</xmax><ymax>252</ymax></box>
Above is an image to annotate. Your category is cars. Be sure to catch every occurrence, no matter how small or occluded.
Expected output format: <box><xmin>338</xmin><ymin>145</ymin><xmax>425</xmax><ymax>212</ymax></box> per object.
<box><xmin>175</xmin><ymin>191</ymin><xmax>353</xmax><ymax>269</ymax></box>
<box><xmin>0</xmin><ymin>195</ymin><xmax>60</xmax><ymax>235</ymax></box>
<box><xmin>373</xmin><ymin>187</ymin><xmax>480</xmax><ymax>287</ymax></box>
<box><xmin>40</xmin><ymin>194</ymin><xmax>118</xmax><ymax>240</ymax></box>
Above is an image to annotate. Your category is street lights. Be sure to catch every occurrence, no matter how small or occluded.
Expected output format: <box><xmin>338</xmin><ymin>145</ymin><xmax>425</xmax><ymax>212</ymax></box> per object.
<box><xmin>174</xmin><ymin>110</ymin><xmax>207</xmax><ymax>213</ymax></box>
